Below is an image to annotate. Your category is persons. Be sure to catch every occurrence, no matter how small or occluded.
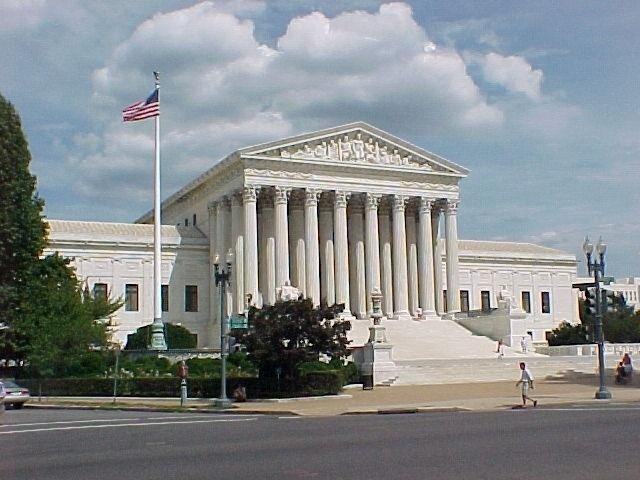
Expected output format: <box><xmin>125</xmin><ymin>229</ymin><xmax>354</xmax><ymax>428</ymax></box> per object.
<box><xmin>521</xmin><ymin>337</ymin><xmax>527</xmax><ymax>354</ymax></box>
<box><xmin>233</xmin><ymin>385</ymin><xmax>247</xmax><ymax>402</ymax></box>
<box><xmin>622</xmin><ymin>353</ymin><xmax>632</xmax><ymax>375</ymax></box>
<box><xmin>499</xmin><ymin>344</ymin><xmax>504</xmax><ymax>360</ymax></box>
<box><xmin>280</xmin><ymin>133</ymin><xmax>433</xmax><ymax>168</ymax></box>
<box><xmin>615</xmin><ymin>362</ymin><xmax>626</xmax><ymax>385</ymax></box>
<box><xmin>498</xmin><ymin>339</ymin><xmax>502</xmax><ymax>353</ymax></box>
<box><xmin>516</xmin><ymin>362</ymin><xmax>537</xmax><ymax>408</ymax></box>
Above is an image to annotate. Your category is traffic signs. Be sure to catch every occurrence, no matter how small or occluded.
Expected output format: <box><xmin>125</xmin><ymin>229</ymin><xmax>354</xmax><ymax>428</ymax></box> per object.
<box><xmin>229</xmin><ymin>315</ymin><xmax>249</xmax><ymax>338</ymax></box>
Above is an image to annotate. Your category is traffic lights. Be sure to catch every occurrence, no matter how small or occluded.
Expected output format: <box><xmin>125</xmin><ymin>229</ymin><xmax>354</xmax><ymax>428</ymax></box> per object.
<box><xmin>584</xmin><ymin>289</ymin><xmax>598</xmax><ymax>315</ymax></box>
<box><xmin>602</xmin><ymin>290</ymin><xmax>614</xmax><ymax>310</ymax></box>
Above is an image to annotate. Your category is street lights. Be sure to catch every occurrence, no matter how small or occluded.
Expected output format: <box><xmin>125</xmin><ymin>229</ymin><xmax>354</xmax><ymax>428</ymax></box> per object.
<box><xmin>581</xmin><ymin>234</ymin><xmax>615</xmax><ymax>400</ymax></box>
<box><xmin>210</xmin><ymin>248</ymin><xmax>237</xmax><ymax>408</ymax></box>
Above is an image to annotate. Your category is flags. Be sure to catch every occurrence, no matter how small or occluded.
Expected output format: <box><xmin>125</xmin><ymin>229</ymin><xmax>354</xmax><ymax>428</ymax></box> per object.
<box><xmin>122</xmin><ymin>88</ymin><xmax>160</xmax><ymax>122</ymax></box>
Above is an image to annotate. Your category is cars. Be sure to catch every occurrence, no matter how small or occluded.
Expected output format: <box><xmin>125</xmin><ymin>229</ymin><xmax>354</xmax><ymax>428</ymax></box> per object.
<box><xmin>0</xmin><ymin>378</ymin><xmax>32</xmax><ymax>410</ymax></box>
